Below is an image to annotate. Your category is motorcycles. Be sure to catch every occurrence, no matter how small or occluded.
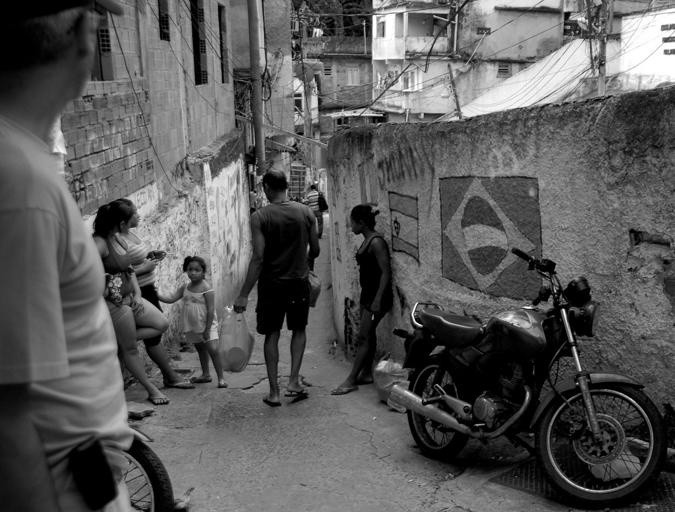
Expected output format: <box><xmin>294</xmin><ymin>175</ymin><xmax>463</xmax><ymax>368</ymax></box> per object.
<box><xmin>385</xmin><ymin>245</ymin><xmax>671</xmax><ymax>504</ymax></box>
<box><xmin>120</xmin><ymin>424</ymin><xmax>175</xmax><ymax>512</ymax></box>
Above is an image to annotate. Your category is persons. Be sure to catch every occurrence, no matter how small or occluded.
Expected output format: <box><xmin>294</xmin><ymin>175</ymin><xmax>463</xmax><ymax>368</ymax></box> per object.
<box><xmin>153</xmin><ymin>255</ymin><xmax>228</xmax><ymax>388</ymax></box>
<box><xmin>306</xmin><ymin>184</ymin><xmax>324</xmax><ymax>239</ymax></box>
<box><xmin>232</xmin><ymin>170</ymin><xmax>323</xmax><ymax>407</ymax></box>
<box><xmin>114</xmin><ymin>196</ymin><xmax>197</xmax><ymax>388</ymax></box>
<box><xmin>0</xmin><ymin>1</ymin><xmax>132</xmax><ymax>510</ymax></box>
<box><xmin>328</xmin><ymin>204</ymin><xmax>394</xmax><ymax>395</ymax></box>
<box><xmin>87</xmin><ymin>200</ymin><xmax>172</xmax><ymax>405</ymax></box>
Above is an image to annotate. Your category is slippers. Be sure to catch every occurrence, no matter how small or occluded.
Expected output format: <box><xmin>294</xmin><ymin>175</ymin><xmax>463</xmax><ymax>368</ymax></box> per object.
<box><xmin>284</xmin><ymin>390</ymin><xmax>307</xmax><ymax>397</ymax></box>
<box><xmin>148</xmin><ymin>396</ymin><xmax>170</xmax><ymax>405</ymax></box>
<box><xmin>164</xmin><ymin>379</ymin><xmax>195</xmax><ymax>389</ymax></box>
<box><xmin>263</xmin><ymin>396</ymin><xmax>281</xmax><ymax>406</ymax></box>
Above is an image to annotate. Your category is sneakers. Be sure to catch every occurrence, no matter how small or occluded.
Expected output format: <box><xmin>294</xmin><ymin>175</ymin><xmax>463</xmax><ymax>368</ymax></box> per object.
<box><xmin>218</xmin><ymin>383</ymin><xmax>228</xmax><ymax>388</ymax></box>
<box><xmin>331</xmin><ymin>371</ymin><xmax>373</xmax><ymax>395</ymax></box>
<box><xmin>191</xmin><ymin>376</ymin><xmax>212</xmax><ymax>383</ymax></box>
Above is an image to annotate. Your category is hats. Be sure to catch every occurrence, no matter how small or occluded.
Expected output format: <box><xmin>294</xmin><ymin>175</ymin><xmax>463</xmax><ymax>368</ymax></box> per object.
<box><xmin>0</xmin><ymin>0</ymin><xmax>123</xmax><ymax>16</ymax></box>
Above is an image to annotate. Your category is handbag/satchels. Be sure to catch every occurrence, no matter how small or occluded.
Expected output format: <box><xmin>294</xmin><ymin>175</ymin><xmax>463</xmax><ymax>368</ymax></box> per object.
<box><xmin>318</xmin><ymin>192</ymin><xmax>328</xmax><ymax>212</ymax></box>
